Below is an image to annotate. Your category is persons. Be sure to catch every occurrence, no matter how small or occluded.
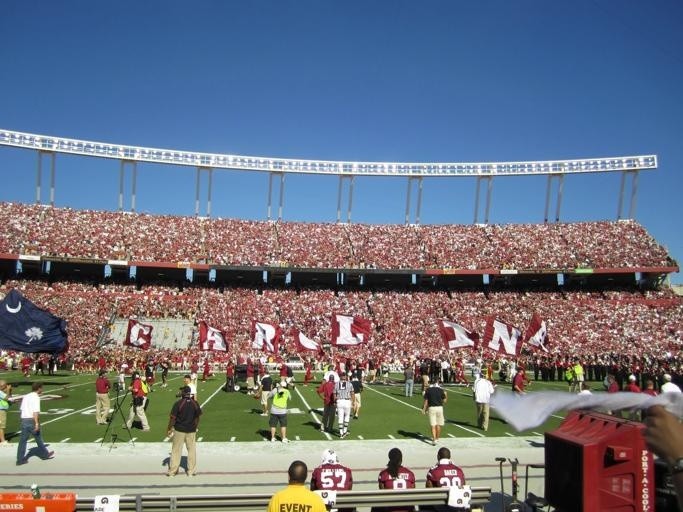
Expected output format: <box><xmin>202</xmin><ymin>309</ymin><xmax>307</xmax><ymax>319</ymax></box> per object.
<box><xmin>13</xmin><ymin>383</ymin><xmax>53</xmax><ymax>465</ymax></box>
<box><xmin>307</xmin><ymin>448</ymin><xmax>356</xmax><ymax>512</ymax></box>
<box><xmin>418</xmin><ymin>447</ymin><xmax>471</xmax><ymax>512</ymax></box>
<box><xmin>266</xmin><ymin>461</ymin><xmax>328</xmax><ymax>512</ymax></box>
<box><xmin>1</xmin><ymin>358</ymin><xmax>497</xmax><ymax>445</ymax></box>
<box><xmin>642</xmin><ymin>405</ymin><xmax>683</xmax><ymax>510</ymax></box>
<box><xmin>2</xmin><ymin>200</ymin><xmax>682</xmax><ymax>398</ymax></box>
<box><xmin>370</xmin><ymin>448</ymin><xmax>416</xmax><ymax>512</ymax></box>
<box><xmin>167</xmin><ymin>386</ymin><xmax>202</xmax><ymax>476</ymax></box>
<box><xmin>671</xmin><ymin>458</ymin><xmax>683</xmax><ymax>475</ymax></box>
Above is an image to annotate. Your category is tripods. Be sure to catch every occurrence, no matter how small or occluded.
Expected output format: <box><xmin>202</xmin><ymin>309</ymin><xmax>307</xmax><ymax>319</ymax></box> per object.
<box><xmin>98</xmin><ymin>403</ymin><xmax>135</xmax><ymax>450</ymax></box>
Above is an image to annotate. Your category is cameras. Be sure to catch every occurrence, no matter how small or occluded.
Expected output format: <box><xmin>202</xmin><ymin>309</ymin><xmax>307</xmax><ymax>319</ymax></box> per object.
<box><xmin>6</xmin><ymin>382</ymin><xmax>18</xmax><ymax>389</ymax></box>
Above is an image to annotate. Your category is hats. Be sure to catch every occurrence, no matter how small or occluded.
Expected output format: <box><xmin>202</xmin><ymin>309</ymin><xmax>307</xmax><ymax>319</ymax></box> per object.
<box><xmin>180</xmin><ymin>387</ymin><xmax>190</xmax><ymax>393</ymax></box>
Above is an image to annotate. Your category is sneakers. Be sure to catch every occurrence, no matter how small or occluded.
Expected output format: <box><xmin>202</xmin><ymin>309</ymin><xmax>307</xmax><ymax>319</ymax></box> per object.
<box><xmin>336</xmin><ymin>431</ymin><xmax>348</xmax><ymax>438</ymax></box>
<box><xmin>97</xmin><ymin>421</ymin><xmax>107</xmax><ymax>424</ymax></box>
<box><xmin>43</xmin><ymin>451</ymin><xmax>54</xmax><ymax>459</ymax></box>
<box><xmin>17</xmin><ymin>460</ymin><xmax>28</xmax><ymax>465</ymax></box>
<box><xmin>123</xmin><ymin>424</ymin><xmax>150</xmax><ymax>431</ymax></box>
<box><xmin>272</xmin><ymin>438</ymin><xmax>288</xmax><ymax>443</ymax></box>
<box><xmin>320</xmin><ymin>428</ymin><xmax>331</xmax><ymax>432</ymax></box>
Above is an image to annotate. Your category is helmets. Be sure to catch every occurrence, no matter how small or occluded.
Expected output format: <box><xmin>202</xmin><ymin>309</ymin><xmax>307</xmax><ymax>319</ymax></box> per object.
<box><xmin>322</xmin><ymin>450</ymin><xmax>339</xmax><ymax>465</ymax></box>
